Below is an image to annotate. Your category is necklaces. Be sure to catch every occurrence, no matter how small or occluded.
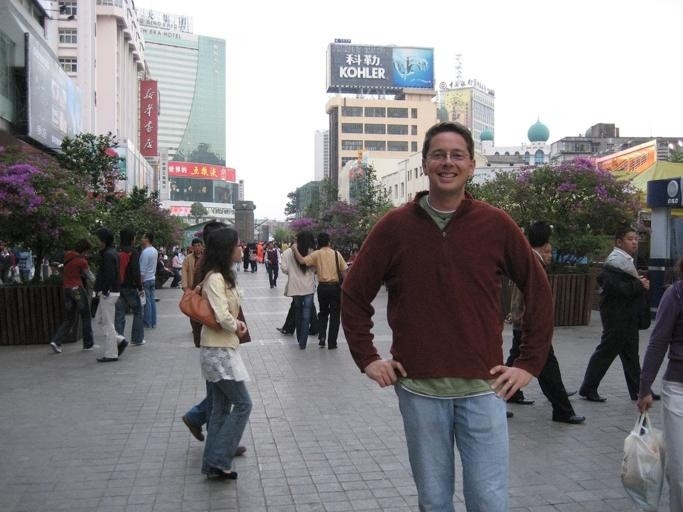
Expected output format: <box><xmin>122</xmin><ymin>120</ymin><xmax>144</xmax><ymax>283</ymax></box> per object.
<box><xmin>426</xmin><ymin>204</ymin><xmax>454</xmax><ymax>221</ymax></box>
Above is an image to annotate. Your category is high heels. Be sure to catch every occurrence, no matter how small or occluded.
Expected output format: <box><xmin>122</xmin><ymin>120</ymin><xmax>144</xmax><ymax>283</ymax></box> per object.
<box><xmin>206</xmin><ymin>466</ymin><xmax>238</xmax><ymax>480</ymax></box>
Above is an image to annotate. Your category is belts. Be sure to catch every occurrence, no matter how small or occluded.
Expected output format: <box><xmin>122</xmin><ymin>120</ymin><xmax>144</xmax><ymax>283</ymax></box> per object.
<box><xmin>318</xmin><ymin>281</ymin><xmax>338</xmax><ymax>287</ymax></box>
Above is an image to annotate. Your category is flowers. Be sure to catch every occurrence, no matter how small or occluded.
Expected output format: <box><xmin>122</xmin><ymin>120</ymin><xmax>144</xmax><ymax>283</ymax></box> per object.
<box><xmin>0</xmin><ymin>140</ymin><xmax>110</xmax><ymax>285</ymax></box>
<box><xmin>471</xmin><ymin>152</ymin><xmax>646</xmax><ymax>275</ymax></box>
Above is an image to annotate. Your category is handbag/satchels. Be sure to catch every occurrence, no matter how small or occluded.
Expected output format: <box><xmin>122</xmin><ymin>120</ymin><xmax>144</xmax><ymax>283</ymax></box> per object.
<box><xmin>179</xmin><ymin>271</ymin><xmax>224</xmax><ymax>332</ymax></box>
<box><xmin>621</xmin><ymin>407</ymin><xmax>667</xmax><ymax>512</ymax></box>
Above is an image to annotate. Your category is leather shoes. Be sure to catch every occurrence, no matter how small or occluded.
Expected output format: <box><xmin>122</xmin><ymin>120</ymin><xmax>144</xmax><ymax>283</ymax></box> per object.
<box><xmin>277</xmin><ymin>327</ymin><xmax>295</xmax><ymax>335</ymax></box>
<box><xmin>318</xmin><ymin>337</ymin><xmax>325</xmax><ymax>346</ymax></box>
<box><xmin>515</xmin><ymin>397</ymin><xmax>535</xmax><ymax>405</ymax></box>
<box><xmin>579</xmin><ymin>389</ymin><xmax>608</xmax><ymax>402</ymax></box>
<box><xmin>630</xmin><ymin>390</ymin><xmax>662</xmax><ymax>401</ymax></box>
<box><xmin>328</xmin><ymin>341</ymin><xmax>338</xmax><ymax>349</ymax></box>
<box><xmin>552</xmin><ymin>414</ymin><xmax>586</xmax><ymax>424</ymax></box>
<box><xmin>565</xmin><ymin>390</ymin><xmax>577</xmax><ymax>397</ymax></box>
<box><xmin>309</xmin><ymin>328</ymin><xmax>318</xmax><ymax>335</ymax></box>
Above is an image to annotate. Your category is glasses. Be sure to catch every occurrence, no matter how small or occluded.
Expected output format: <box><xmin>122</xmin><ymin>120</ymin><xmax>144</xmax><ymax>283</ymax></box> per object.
<box><xmin>424</xmin><ymin>149</ymin><xmax>470</xmax><ymax>161</ymax></box>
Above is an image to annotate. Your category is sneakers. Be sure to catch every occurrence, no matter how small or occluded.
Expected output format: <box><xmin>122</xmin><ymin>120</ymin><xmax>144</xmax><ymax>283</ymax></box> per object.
<box><xmin>183</xmin><ymin>414</ymin><xmax>206</xmax><ymax>441</ymax></box>
<box><xmin>82</xmin><ymin>343</ymin><xmax>100</xmax><ymax>351</ymax></box>
<box><xmin>50</xmin><ymin>341</ymin><xmax>63</xmax><ymax>353</ymax></box>
<box><xmin>234</xmin><ymin>445</ymin><xmax>246</xmax><ymax>456</ymax></box>
<box><xmin>132</xmin><ymin>339</ymin><xmax>146</xmax><ymax>345</ymax></box>
<box><xmin>118</xmin><ymin>338</ymin><xmax>129</xmax><ymax>355</ymax></box>
<box><xmin>96</xmin><ymin>356</ymin><xmax>119</xmax><ymax>362</ymax></box>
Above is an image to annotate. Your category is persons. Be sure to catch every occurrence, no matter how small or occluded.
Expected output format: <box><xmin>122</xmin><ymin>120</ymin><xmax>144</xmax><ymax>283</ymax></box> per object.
<box><xmin>500</xmin><ymin>220</ymin><xmax>587</xmax><ymax>425</ymax></box>
<box><xmin>577</xmin><ymin>225</ymin><xmax>661</xmax><ymax>402</ymax></box>
<box><xmin>178</xmin><ymin>220</ymin><xmax>248</xmax><ymax>459</ymax></box>
<box><xmin>198</xmin><ymin>228</ymin><xmax>253</xmax><ymax>485</ymax></box>
<box><xmin>635</xmin><ymin>254</ymin><xmax>683</xmax><ymax>512</ymax></box>
<box><xmin>507</xmin><ymin>240</ymin><xmax>578</xmax><ymax>405</ymax></box>
<box><xmin>336</xmin><ymin>120</ymin><xmax>558</xmax><ymax>512</ymax></box>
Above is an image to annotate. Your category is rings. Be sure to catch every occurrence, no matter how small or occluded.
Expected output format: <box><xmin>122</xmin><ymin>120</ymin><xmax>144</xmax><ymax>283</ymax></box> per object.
<box><xmin>507</xmin><ymin>378</ymin><xmax>512</xmax><ymax>385</ymax></box>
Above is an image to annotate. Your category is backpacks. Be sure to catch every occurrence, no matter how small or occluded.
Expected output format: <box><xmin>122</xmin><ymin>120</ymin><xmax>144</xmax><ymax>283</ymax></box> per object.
<box><xmin>118</xmin><ymin>249</ymin><xmax>132</xmax><ymax>284</ymax></box>
